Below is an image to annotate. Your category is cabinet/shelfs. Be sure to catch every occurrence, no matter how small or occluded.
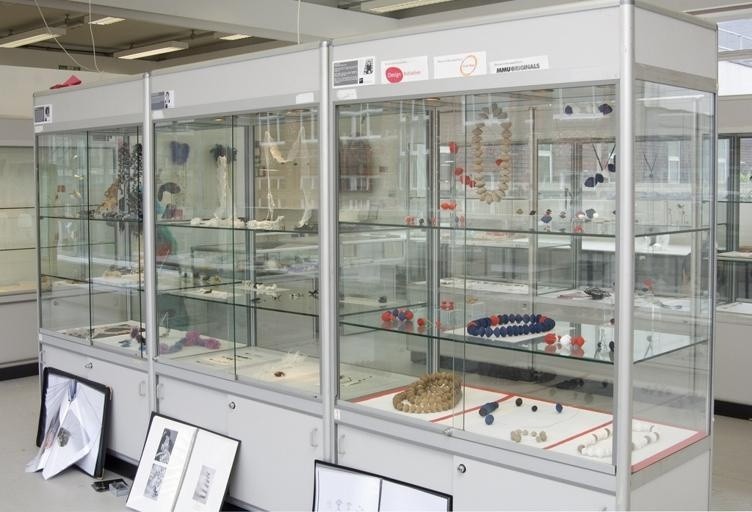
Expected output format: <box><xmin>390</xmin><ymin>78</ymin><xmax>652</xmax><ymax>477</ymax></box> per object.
<box><xmin>328</xmin><ymin>1</ymin><xmax>719</xmax><ymax>511</ymax></box>
<box><xmin>0</xmin><ymin>113</ymin><xmax>39</xmax><ymax>380</ymax></box>
<box><xmin>145</xmin><ymin>39</ymin><xmax>330</xmax><ymax>512</ymax></box>
<box><xmin>34</xmin><ymin>75</ymin><xmax>149</xmax><ymax>481</ymax></box>
<box><xmin>711</xmin><ymin>93</ymin><xmax>752</xmax><ymax>408</ymax></box>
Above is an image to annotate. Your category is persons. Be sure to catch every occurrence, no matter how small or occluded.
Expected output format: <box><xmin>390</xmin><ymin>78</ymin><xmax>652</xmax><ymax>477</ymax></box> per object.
<box><xmin>154</xmin><ymin>432</ymin><xmax>174</xmax><ymax>465</ymax></box>
